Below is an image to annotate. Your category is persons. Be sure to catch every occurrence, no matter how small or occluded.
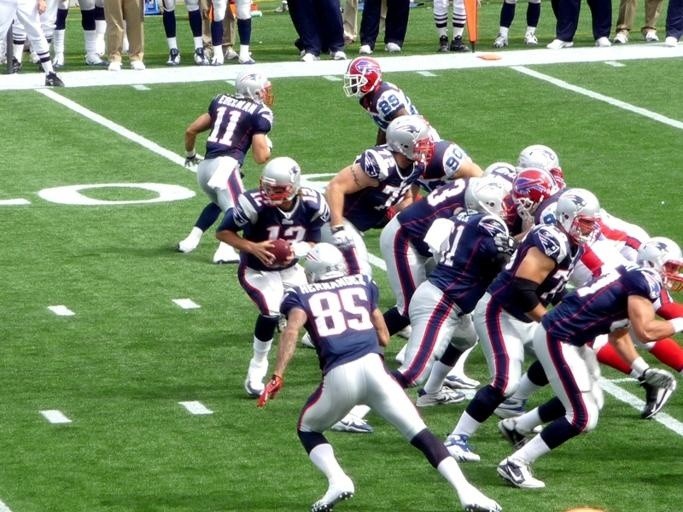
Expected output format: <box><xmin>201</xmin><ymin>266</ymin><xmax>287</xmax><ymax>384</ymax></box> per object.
<box><xmin>433</xmin><ymin>0</ymin><xmax>683</xmax><ymax>53</ymax></box>
<box><xmin>341</xmin><ymin>55</ymin><xmax>439</xmax><ymax>147</ymax></box>
<box><xmin>162</xmin><ymin>0</ymin><xmax>255</xmax><ymax>64</ymax></box>
<box><xmin>257</xmin><ymin>244</ymin><xmax>503</xmax><ymax>509</ymax></box>
<box><xmin>173</xmin><ymin>72</ymin><xmax>276</xmax><ymax>266</ymax></box>
<box><xmin>287</xmin><ymin>0</ymin><xmax>411</xmax><ymax>62</ymax></box>
<box><xmin>1</xmin><ymin>0</ymin><xmax>146</xmax><ymax>89</ymax></box>
<box><xmin>215</xmin><ymin>157</ymin><xmax>331</xmax><ymax>397</ymax></box>
<box><xmin>326</xmin><ymin>113</ymin><xmax>681</xmax><ymax>510</ymax></box>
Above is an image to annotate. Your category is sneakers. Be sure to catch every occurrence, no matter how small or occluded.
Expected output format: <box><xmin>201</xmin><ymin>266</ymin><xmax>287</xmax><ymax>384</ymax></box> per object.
<box><xmin>665</xmin><ymin>35</ymin><xmax>677</xmax><ymax>47</ymax></box>
<box><xmin>274</xmin><ymin>316</ymin><xmax>315</xmax><ymax>349</ymax></box>
<box><xmin>0</xmin><ymin>52</ymin><xmax>65</xmax><ymax>69</ymax></box>
<box><xmin>312</xmin><ymin>477</ymin><xmax>354</xmax><ymax>512</ymax></box>
<box><xmin>331</xmin><ymin>415</ymin><xmax>372</xmax><ymax>433</ymax></box>
<box><xmin>613</xmin><ymin>30</ymin><xmax>628</xmax><ymax>44</ymax></box>
<box><xmin>396</xmin><ymin>325</ymin><xmax>545</xmax><ymax>512</ymax></box>
<box><xmin>211</xmin><ymin>248</ymin><xmax>239</xmax><ymax>264</ymax></box>
<box><xmin>547</xmin><ymin>39</ymin><xmax>574</xmax><ymax>49</ymax></box>
<box><xmin>438</xmin><ymin>35</ymin><xmax>470</xmax><ymax>52</ymax></box>
<box><xmin>638</xmin><ymin>376</ymin><xmax>676</xmax><ymax>420</ymax></box>
<box><xmin>244</xmin><ymin>357</ymin><xmax>269</xmax><ymax>394</ymax></box>
<box><xmin>300</xmin><ymin>37</ymin><xmax>402</xmax><ymax>61</ymax></box>
<box><xmin>596</xmin><ymin>38</ymin><xmax>612</xmax><ymax>47</ymax></box>
<box><xmin>45</xmin><ymin>72</ymin><xmax>64</xmax><ymax>87</ymax></box>
<box><xmin>645</xmin><ymin>31</ymin><xmax>659</xmax><ymax>42</ymax></box>
<box><xmin>167</xmin><ymin>45</ymin><xmax>256</xmax><ymax>65</ymax></box>
<box><xmin>524</xmin><ymin>32</ymin><xmax>537</xmax><ymax>46</ymax></box>
<box><xmin>177</xmin><ymin>240</ymin><xmax>195</xmax><ymax>253</ymax></box>
<box><xmin>494</xmin><ymin>32</ymin><xmax>509</xmax><ymax>47</ymax></box>
<box><xmin>85</xmin><ymin>53</ymin><xmax>145</xmax><ymax>70</ymax></box>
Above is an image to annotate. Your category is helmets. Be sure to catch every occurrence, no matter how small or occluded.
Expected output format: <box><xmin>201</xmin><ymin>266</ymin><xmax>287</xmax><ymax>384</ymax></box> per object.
<box><xmin>305</xmin><ymin>242</ymin><xmax>348</xmax><ymax>282</ymax></box>
<box><xmin>235</xmin><ymin>72</ymin><xmax>274</xmax><ymax>106</ymax></box>
<box><xmin>556</xmin><ymin>188</ymin><xmax>603</xmax><ymax>246</ymax></box>
<box><xmin>638</xmin><ymin>236</ymin><xmax>682</xmax><ymax>293</ymax></box>
<box><xmin>464</xmin><ymin>144</ymin><xmax>561</xmax><ymax>221</ymax></box>
<box><xmin>258</xmin><ymin>156</ymin><xmax>301</xmax><ymax>206</ymax></box>
<box><xmin>385</xmin><ymin>115</ymin><xmax>434</xmax><ymax>166</ymax></box>
<box><xmin>343</xmin><ymin>56</ymin><xmax>381</xmax><ymax>99</ymax></box>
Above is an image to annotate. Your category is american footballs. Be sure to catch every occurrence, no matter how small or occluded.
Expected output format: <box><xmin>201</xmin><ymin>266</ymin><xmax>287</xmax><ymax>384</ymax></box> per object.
<box><xmin>266</xmin><ymin>239</ymin><xmax>290</xmax><ymax>267</ymax></box>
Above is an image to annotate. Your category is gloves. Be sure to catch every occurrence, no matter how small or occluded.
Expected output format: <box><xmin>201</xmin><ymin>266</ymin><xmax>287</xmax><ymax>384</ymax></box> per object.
<box><xmin>494</xmin><ymin>230</ymin><xmax>519</xmax><ymax>254</ymax></box>
<box><xmin>183</xmin><ymin>151</ymin><xmax>201</xmax><ymax>168</ymax></box>
<box><xmin>332</xmin><ymin>224</ymin><xmax>355</xmax><ymax>251</ymax></box>
<box><xmin>257</xmin><ymin>374</ymin><xmax>283</xmax><ymax>407</ymax></box>
<box><xmin>643</xmin><ymin>366</ymin><xmax>676</xmax><ymax>392</ymax></box>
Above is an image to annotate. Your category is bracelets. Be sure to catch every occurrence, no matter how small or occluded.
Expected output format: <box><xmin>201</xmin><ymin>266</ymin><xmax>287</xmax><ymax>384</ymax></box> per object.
<box><xmin>184</xmin><ymin>148</ymin><xmax>196</xmax><ymax>158</ymax></box>
